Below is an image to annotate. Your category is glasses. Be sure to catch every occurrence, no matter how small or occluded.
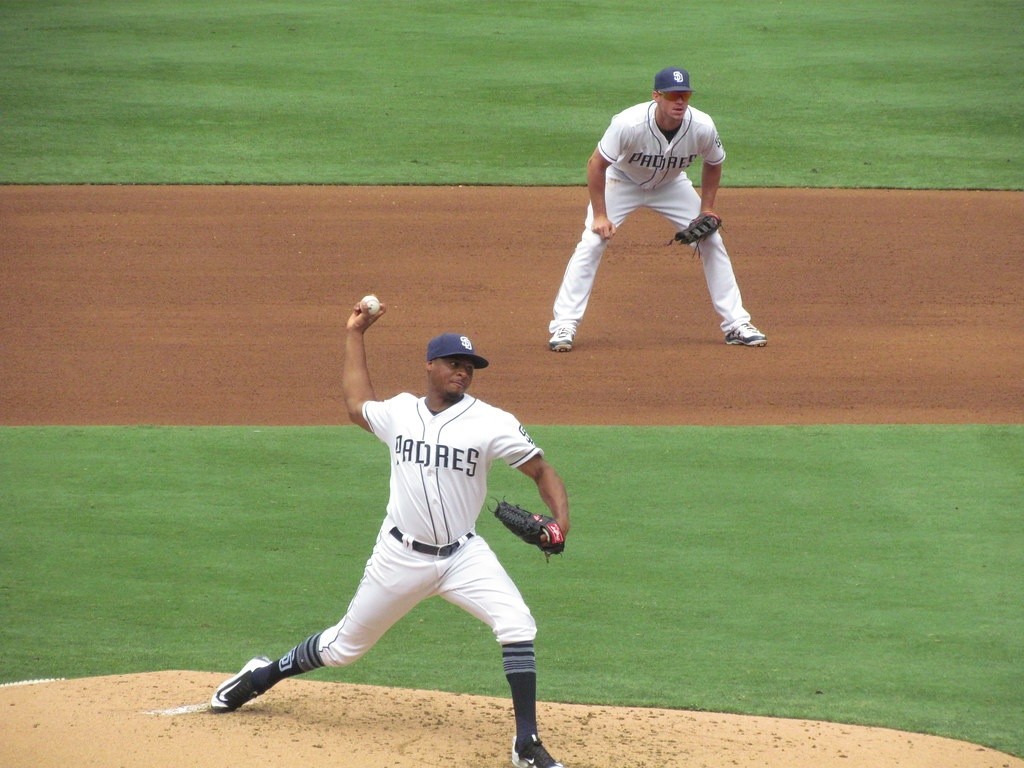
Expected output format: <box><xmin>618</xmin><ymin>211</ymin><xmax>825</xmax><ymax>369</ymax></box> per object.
<box><xmin>659</xmin><ymin>92</ymin><xmax>692</xmax><ymax>102</ymax></box>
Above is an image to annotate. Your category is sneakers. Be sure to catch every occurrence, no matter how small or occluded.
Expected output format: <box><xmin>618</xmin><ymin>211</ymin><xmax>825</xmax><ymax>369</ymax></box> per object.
<box><xmin>511</xmin><ymin>735</ymin><xmax>564</xmax><ymax>768</ymax></box>
<box><xmin>725</xmin><ymin>321</ymin><xmax>768</xmax><ymax>346</ymax></box>
<box><xmin>211</xmin><ymin>655</ymin><xmax>274</xmax><ymax>713</ymax></box>
<box><xmin>548</xmin><ymin>328</ymin><xmax>574</xmax><ymax>351</ymax></box>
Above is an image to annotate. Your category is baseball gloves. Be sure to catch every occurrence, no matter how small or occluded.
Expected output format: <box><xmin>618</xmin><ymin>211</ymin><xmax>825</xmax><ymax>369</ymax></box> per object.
<box><xmin>667</xmin><ymin>210</ymin><xmax>729</xmax><ymax>260</ymax></box>
<box><xmin>487</xmin><ymin>494</ymin><xmax>566</xmax><ymax>565</ymax></box>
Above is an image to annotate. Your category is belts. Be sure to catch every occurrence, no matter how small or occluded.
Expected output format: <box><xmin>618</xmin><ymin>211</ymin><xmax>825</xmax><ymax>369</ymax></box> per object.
<box><xmin>388</xmin><ymin>528</ymin><xmax>473</xmax><ymax>556</ymax></box>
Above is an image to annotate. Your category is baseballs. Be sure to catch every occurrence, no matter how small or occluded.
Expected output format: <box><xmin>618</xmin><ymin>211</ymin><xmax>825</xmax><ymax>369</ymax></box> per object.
<box><xmin>359</xmin><ymin>294</ymin><xmax>381</xmax><ymax>316</ymax></box>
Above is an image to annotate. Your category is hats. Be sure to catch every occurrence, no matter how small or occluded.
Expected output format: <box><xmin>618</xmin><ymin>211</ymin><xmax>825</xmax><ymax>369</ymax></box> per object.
<box><xmin>654</xmin><ymin>66</ymin><xmax>695</xmax><ymax>93</ymax></box>
<box><xmin>427</xmin><ymin>333</ymin><xmax>489</xmax><ymax>369</ymax></box>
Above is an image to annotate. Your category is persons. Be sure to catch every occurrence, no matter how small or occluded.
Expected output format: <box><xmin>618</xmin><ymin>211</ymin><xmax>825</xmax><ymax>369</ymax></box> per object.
<box><xmin>211</xmin><ymin>293</ymin><xmax>571</xmax><ymax>768</ymax></box>
<box><xmin>549</xmin><ymin>66</ymin><xmax>767</xmax><ymax>351</ymax></box>
